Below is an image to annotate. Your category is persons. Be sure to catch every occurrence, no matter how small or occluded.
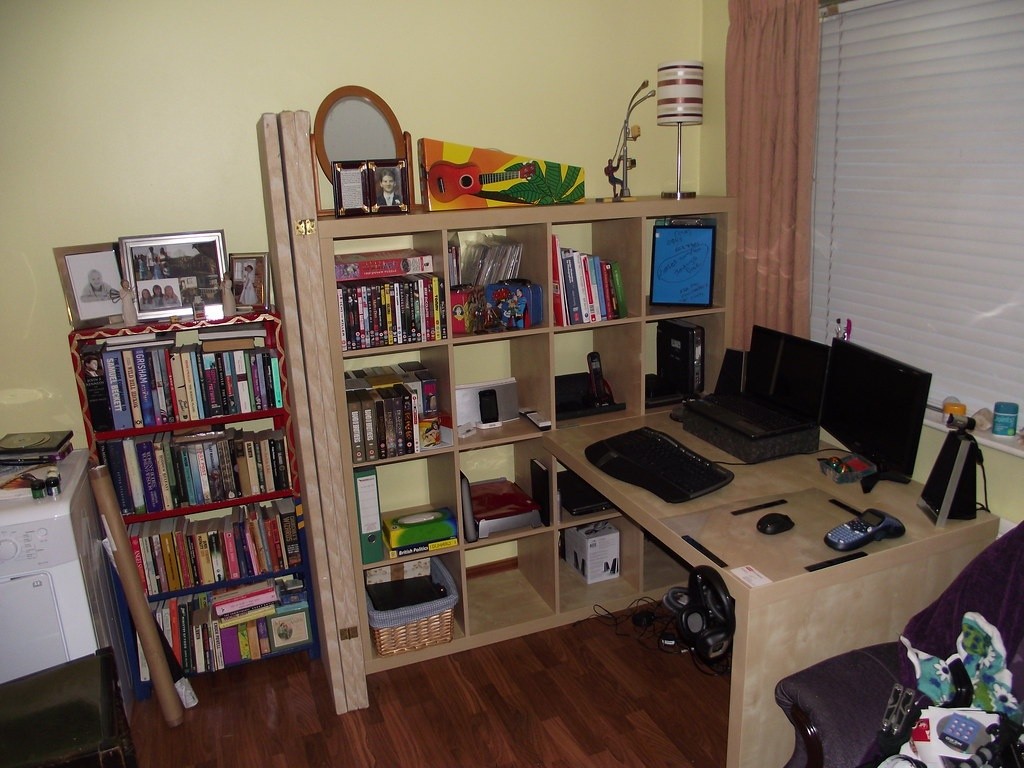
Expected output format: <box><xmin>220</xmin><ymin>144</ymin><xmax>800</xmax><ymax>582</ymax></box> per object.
<box><xmin>79</xmin><ymin>270</ymin><xmax>118</xmax><ymax>301</ymax></box>
<box><xmin>119</xmin><ymin>279</ymin><xmax>139</xmax><ymax>326</ymax></box>
<box><xmin>604</xmin><ymin>156</ymin><xmax>624</xmax><ymax>198</ymax></box>
<box><xmin>140</xmin><ymin>284</ymin><xmax>180</xmax><ymax>310</ymax></box>
<box><xmin>376</xmin><ymin>168</ymin><xmax>402</xmax><ymax>206</ymax></box>
<box><xmin>240</xmin><ymin>265</ymin><xmax>261</xmax><ymax>306</ymax></box>
<box><xmin>138</xmin><ymin>246</ymin><xmax>170</xmax><ymax>280</ymax></box>
<box><xmin>220</xmin><ymin>271</ymin><xmax>236</xmax><ymax>316</ymax></box>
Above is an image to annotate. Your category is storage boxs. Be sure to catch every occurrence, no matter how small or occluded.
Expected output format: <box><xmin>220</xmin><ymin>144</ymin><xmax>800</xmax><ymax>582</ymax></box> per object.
<box><xmin>564</xmin><ymin>521</ymin><xmax>620</xmax><ymax>586</ymax></box>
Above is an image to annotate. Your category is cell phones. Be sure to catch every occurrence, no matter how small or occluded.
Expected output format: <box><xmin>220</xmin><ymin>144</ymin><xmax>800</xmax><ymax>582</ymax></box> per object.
<box><xmin>478</xmin><ymin>389</ymin><xmax>499</xmax><ymax>423</ymax></box>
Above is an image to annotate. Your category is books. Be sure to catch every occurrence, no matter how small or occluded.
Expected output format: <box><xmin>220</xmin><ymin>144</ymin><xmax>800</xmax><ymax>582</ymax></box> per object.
<box><xmin>126</xmin><ymin>497</ymin><xmax>303</xmax><ymax>595</ymax></box>
<box><xmin>333</xmin><ymin>247</ymin><xmax>448</xmax><ymax>352</ymax></box>
<box><xmin>447</xmin><ymin>236</ymin><xmax>522</xmax><ymax>287</ymax></box>
<box><xmin>551</xmin><ymin>232</ymin><xmax>629</xmax><ymax>328</ymax></box>
<box><xmin>80</xmin><ymin>322</ymin><xmax>282</xmax><ymax>433</ymax></box>
<box><xmin>529</xmin><ymin>457</ymin><xmax>552</xmax><ymax>527</ymax></box>
<box><xmin>137</xmin><ymin>575</ymin><xmax>306</xmax><ymax>681</ymax></box>
<box><xmin>94</xmin><ymin>415</ymin><xmax>293</xmax><ymax>515</ymax></box>
<box><xmin>344</xmin><ymin>362</ymin><xmax>439</xmax><ymax>463</ymax></box>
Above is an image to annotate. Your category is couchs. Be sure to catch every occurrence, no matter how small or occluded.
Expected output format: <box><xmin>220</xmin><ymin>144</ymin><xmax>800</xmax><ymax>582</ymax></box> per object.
<box><xmin>774</xmin><ymin>516</ymin><xmax>1024</xmax><ymax>768</ymax></box>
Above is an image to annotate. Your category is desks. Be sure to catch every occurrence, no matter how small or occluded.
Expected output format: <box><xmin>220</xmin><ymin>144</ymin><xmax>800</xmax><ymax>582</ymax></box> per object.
<box><xmin>541</xmin><ymin>408</ymin><xmax>999</xmax><ymax>768</ymax></box>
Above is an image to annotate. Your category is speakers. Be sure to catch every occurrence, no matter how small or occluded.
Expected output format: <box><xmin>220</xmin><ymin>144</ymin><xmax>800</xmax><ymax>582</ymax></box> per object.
<box><xmin>713</xmin><ymin>347</ymin><xmax>749</xmax><ymax>395</ymax></box>
<box><xmin>916</xmin><ymin>430</ymin><xmax>976</xmax><ymax>528</ymax></box>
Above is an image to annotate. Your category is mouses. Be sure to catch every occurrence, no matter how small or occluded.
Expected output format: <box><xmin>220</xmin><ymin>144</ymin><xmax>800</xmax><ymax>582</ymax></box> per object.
<box><xmin>756</xmin><ymin>513</ymin><xmax>794</xmax><ymax>534</ymax></box>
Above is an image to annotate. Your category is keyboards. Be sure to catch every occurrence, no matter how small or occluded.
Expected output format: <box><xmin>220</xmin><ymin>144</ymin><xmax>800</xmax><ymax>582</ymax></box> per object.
<box><xmin>585</xmin><ymin>426</ymin><xmax>734</xmax><ymax>503</ymax></box>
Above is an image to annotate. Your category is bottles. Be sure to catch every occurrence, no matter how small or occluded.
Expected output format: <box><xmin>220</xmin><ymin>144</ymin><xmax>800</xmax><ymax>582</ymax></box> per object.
<box><xmin>31</xmin><ymin>466</ymin><xmax>61</xmax><ymax>499</ymax></box>
<box><xmin>941</xmin><ymin>402</ymin><xmax>966</xmax><ymax>425</ymax></box>
<box><xmin>992</xmin><ymin>401</ymin><xmax>1019</xmax><ymax>437</ymax></box>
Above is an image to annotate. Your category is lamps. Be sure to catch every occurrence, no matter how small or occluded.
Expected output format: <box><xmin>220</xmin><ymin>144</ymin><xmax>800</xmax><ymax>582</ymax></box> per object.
<box><xmin>653</xmin><ymin>60</ymin><xmax>705</xmax><ymax>198</ymax></box>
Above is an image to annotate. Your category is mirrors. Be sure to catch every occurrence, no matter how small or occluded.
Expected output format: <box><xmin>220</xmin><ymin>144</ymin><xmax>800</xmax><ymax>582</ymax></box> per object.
<box><xmin>310</xmin><ymin>83</ymin><xmax>414</xmax><ymax>217</ymax></box>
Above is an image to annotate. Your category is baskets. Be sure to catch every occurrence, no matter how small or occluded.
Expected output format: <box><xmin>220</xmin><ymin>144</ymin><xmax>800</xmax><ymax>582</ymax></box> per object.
<box><xmin>363</xmin><ymin>557</ymin><xmax>459</xmax><ymax>657</ymax></box>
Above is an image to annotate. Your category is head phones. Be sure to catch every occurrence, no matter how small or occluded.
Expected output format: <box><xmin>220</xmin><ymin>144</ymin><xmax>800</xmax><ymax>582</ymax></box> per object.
<box><xmin>677</xmin><ymin>564</ymin><xmax>736</xmax><ymax>665</ymax></box>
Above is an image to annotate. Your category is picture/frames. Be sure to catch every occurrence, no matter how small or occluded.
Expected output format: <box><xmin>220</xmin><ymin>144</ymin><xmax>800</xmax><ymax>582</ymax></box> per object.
<box><xmin>228</xmin><ymin>251</ymin><xmax>272</xmax><ymax>314</ymax></box>
<box><xmin>52</xmin><ymin>243</ymin><xmax>126</xmax><ymax>332</ymax></box>
<box><xmin>119</xmin><ymin>229</ymin><xmax>230</xmax><ymax>323</ymax></box>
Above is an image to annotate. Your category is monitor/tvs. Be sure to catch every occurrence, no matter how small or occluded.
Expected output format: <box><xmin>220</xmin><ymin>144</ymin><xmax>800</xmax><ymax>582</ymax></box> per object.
<box><xmin>820</xmin><ymin>337</ymin><xmax>932</xmax><ymax>494</ymax></box>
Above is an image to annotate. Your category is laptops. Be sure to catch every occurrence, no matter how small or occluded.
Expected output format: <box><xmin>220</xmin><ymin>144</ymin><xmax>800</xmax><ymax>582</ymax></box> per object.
<box><xmin>686</xmin><ymin>324</ymin><xmax>831</xmax><ymax>439</ymax></box>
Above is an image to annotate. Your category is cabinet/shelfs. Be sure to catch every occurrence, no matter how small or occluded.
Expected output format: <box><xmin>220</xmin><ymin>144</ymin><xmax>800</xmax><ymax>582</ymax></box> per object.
<box><xmin>68</xmin><ymin>311</ymin><xmax>314</xmax><ymax>704</ymax></box>
<box><xmin>318</xmin><ymin>194</ymin><xmax>739</xmax><ymax>678</ymax></box>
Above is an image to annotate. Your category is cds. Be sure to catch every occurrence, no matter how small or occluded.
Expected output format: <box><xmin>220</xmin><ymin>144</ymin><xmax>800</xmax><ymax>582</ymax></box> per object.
<box><xmin>0</xmin><ymin>433</ymin><xmax>50</xmax><ymax>448</ymax></box>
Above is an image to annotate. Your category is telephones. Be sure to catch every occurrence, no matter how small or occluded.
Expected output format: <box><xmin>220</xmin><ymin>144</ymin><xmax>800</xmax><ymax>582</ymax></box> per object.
<box><xmin>588</xmin><ymin>351</ymin><xmax>611</xmax><ymax>409</ymax></box>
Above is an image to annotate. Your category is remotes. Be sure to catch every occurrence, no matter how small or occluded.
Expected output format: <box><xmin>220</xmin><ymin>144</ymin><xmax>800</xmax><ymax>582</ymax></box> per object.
<box><xmin>527</xmin><ymin>412</ymin><xmax>552</xmax><ymax>430</ymax></box>
